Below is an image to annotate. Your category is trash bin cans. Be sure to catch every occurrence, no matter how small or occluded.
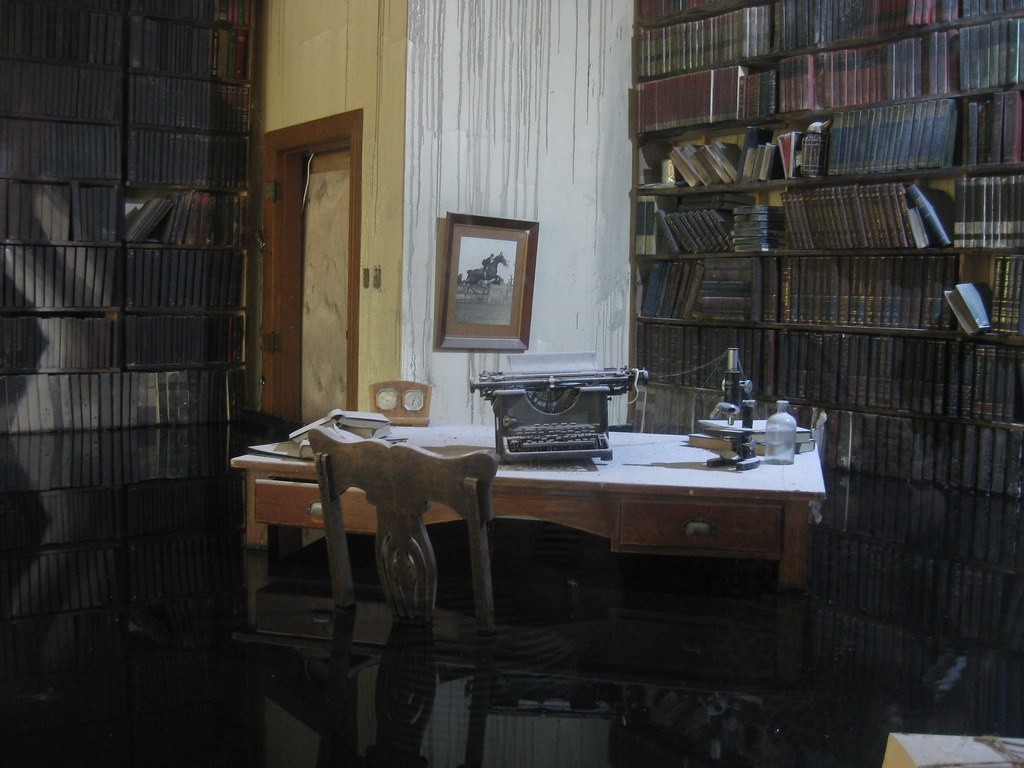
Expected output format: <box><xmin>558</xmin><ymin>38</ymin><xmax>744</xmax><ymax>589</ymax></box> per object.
<box><xmin>488</xmin><ymin>663</ymin><xmax>615</xmax><ymax>767</ymax></box>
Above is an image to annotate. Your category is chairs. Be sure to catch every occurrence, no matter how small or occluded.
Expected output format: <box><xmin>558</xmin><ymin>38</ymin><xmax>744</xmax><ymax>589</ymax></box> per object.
<box><xmin>316</xmin><ymin>640</ymin><xmax>495</xmax><ymax>768</ymax></box>
<box><xmin>309</xmin><ymin>427</ymin><xmax>497</xmax><ymax>632</ymax></box>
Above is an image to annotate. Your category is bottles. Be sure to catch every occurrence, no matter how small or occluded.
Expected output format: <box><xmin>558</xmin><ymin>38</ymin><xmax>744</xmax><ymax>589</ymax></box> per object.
<box><xmin>765</xmin><ymin>400</ymin><xmax>797</xmax><ymax>464</ymax></box>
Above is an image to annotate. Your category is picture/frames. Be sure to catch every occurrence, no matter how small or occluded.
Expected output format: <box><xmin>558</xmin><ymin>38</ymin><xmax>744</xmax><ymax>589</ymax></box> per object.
<box><xmin>434</xmin><ymin>211</ymin><xmax>541</xmax><ymax>351</ymax></box>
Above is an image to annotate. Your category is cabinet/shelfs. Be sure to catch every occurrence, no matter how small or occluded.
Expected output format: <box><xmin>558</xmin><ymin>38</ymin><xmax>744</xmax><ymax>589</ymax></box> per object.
<box><xmin>0</xmin><ymin>0</ymin><xmax>264</xmax><ymax>436</ymax></box>
<box><xmin>627</xmin><ymin>0</ymin><xmax>1024</xmax><ymax>500</ymax></box>
<box><xmin>807</xmin><ymin>466</ymin><xmax>1024</xmax><ymax>768</ymax></box>
<box><xmin>0</xmin><ymin>423</ymin><xmax>288</xmax><ymax>768</ymax></box>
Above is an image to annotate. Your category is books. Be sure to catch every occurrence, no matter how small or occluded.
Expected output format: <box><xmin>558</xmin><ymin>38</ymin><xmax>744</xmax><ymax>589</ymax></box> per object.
<box><xmin>0</xmin><ymin>0</ymin><xmax>252</xmax><ymax>768</ymax></box>
<box><xmin>288</xmin><ymin>408</ymin><xmax>392</xmax><ymax>460</ymax></box>
<box><xmin>628</xmin><ymin>0</ymin><xmax>1024</xmax><ymax>736</ymax></box>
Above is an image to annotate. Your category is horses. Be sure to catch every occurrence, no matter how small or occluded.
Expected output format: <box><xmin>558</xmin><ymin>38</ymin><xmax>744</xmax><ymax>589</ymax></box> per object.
<box><xmin>463</xmin><ymin>252</ymin><xmax>507</xmax><ymax>300</ymax></box>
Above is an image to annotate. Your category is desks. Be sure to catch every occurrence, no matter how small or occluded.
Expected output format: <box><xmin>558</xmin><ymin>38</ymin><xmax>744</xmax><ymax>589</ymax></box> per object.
<box><xmin>231</xmin><ymin>539</ymin><xmax>815</xmax><ymax>701</ymax></box>
<box><xmin>231</xmin><ymin>425</ymin><xmax>827</xmax><ymax>596</ymax></box>
